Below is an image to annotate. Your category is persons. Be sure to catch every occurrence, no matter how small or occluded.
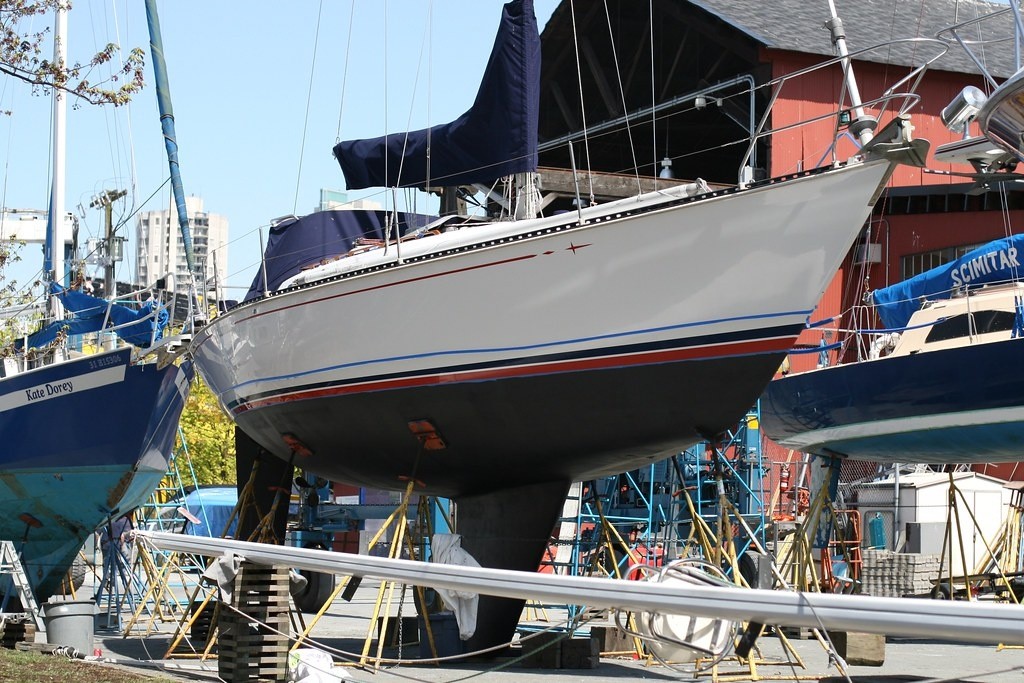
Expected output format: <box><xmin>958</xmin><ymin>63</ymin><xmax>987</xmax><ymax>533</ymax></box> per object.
<box><xmin>94</xmin><ymin>521</ymin><xmax>124</xmax><ymax>580</ymax></box>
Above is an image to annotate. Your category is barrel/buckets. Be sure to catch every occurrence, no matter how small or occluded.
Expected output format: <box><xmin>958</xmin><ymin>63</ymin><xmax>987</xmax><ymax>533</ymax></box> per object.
<box><xmin>40</xmin><ymin>599</ymin><xmax>98</xmax><ymax>658</ymax></box>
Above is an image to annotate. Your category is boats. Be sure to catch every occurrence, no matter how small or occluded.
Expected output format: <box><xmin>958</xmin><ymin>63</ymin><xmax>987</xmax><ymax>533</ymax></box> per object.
<box><xmin>0</xmin><ymin>0</ymin><xmax>1023</xmax><ymax>668</ymax></box>
